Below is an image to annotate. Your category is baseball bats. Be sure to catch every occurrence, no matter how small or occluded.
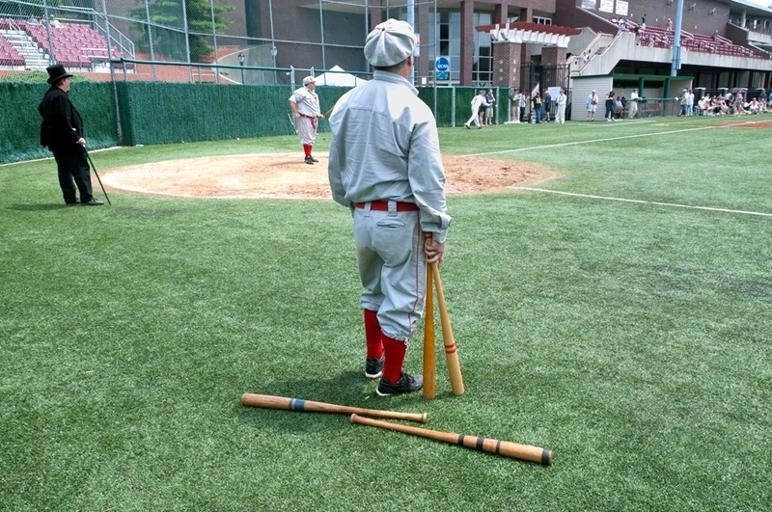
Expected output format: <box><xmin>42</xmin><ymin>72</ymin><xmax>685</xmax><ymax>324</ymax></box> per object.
<box><xmin>432</xmin><ymin>263</ymin><xmax>467</xmax><ymax>396</ymax></box>
<box><xmin>350</xmin><ymin>415</ymin><xmax>553</xmax><ymax>472</ymax></box>
<box><xmin>242</xmin><ymin>392</ymin><xmax>429</xmax><ymax>421</ymax></box>
<box><xmin>424</xmin><ymin>233</ymin><xmax>463</xmax><ymax>401</ymax></box>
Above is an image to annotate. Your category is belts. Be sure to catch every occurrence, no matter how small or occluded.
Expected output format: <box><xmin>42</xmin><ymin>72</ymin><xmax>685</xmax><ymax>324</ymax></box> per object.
<box><xmin>355</xmin><ymin>199</ymin><xmax>420</xmax><ymax>211</ymax></box>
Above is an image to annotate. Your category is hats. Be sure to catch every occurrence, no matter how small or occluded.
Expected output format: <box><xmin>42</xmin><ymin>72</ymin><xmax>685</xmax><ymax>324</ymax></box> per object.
<box><xmin>363</xmin><ymin>18</ymin><xmax>417</xmax><ymax>67</ymax></box>
<box><xmin>46</xmin><ymin>64</ymin><xmax>73</xmax><ymax>84</ymax></box>
<box><xmin>303</xmin><ymin>76</ymin><xmax>316</xmax><ymax>84</ymax></box>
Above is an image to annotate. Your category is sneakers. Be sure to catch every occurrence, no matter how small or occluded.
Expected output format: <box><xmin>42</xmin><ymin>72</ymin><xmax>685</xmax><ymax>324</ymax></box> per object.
<box><xmin>375</xmin><ymin>371</ymin><xmax>423</xmax><ymax>397</ymax></box>
<box><xmin>305</xmin><ymin>155</ymin><xmax>319</xmax><ymax>165</ymax></box>
<box><xmin>365</xmin><ymin>357</ymin><xmax>384</xmax><ymax>379</ymax></box>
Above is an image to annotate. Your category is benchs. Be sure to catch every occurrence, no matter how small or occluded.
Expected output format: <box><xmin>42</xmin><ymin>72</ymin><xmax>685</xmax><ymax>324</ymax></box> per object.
<box><xmin>622</xmin><ymin>103</ymin><xmax>661</xmax><ymax>117</ymax></box>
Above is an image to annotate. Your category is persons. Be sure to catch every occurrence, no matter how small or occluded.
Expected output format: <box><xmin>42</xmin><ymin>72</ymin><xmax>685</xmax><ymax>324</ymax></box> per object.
<box><xmin>325</xmin><ymin>19</ymin><xmax>453</xmax><ymax>399</ymax></box>
<box><xmin>462</xmin><ymin>86</ymin><xmax>643</xmax><ymax>129</ymax></box>
<box><xmin>289</xmin><ymin>75</ymin><xmax>327</xmax><ymax>166</ymax></box>
<box><xmin>36</xmin><ymin>65</ymin><xmax>105</xmax><ymax>208</ymax></box>
<box><xmin>674</xmin><ymin>85</ymin><xmax>772</xmax><ymax>118</ymax></box>
<box><xmin>607</xmin><ymin>13</ymin><xmax>674</xmax><ymax>47</ymax></box>
<box><xmin>712</xmin><ymin>28</ymin><xmax>719</xmax><ymax>41</ymax></box>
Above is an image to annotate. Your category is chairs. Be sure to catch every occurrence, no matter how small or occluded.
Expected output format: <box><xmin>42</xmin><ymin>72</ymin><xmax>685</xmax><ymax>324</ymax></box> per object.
<box><xmin>0</xmin><ymin>18</ymin><xmax>134</xmax><ymax>69</ymax></box>
<box><xmin>611</xmin><ymin>20</ymin><xmax>762</xmax><ymax>57</ymax></box>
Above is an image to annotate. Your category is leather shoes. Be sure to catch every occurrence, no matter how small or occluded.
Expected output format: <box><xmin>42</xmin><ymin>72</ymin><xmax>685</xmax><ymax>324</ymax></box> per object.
<box><xmin>76</xmin><ymin>197</ymin><xmax>79</xmax><ymax>203</ymax></box>
<box><xmin>88</xmin><ymin>198</ymin><xmax>104</xmax><ymax>205</ymax></box>
